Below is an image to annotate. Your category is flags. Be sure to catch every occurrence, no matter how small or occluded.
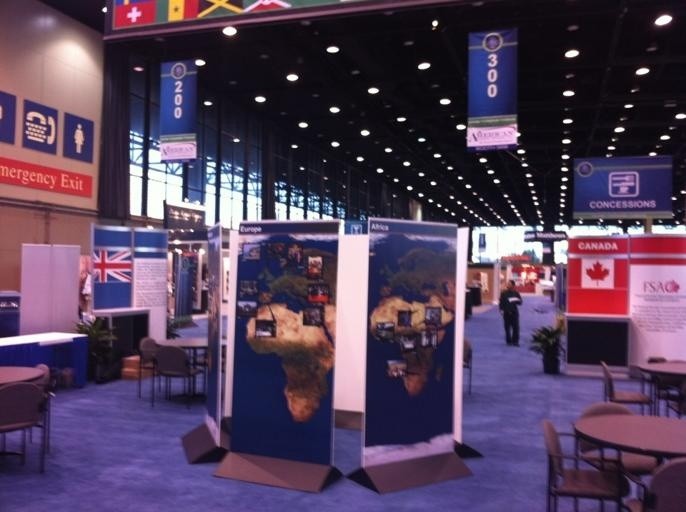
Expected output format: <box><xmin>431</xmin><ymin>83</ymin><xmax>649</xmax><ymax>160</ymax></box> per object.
<box><xmin>93</xmin><ymin>248</ymin><xmax>134</xmax><ymax>284</ymax></box>
<box><xmin>113</xmin><ymin>0</ymin><xmax>345</xmax><ymax>30</ymax></box>
<box><xmin>567</xmin><ymin>258</ymin><xmax>629</xmax><ymax>291</ymax></box>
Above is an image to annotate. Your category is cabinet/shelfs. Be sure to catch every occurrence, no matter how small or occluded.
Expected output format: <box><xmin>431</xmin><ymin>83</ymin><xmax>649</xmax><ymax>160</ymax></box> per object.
<box><xmin>95</xmin><ymin>306</ymin><xmax>152</xmax><ymax>358</ymax></box>
<box><xmin>0</xmin><ymin>290</ymin><xmax>21</xmax><ymax>338</ymax></box>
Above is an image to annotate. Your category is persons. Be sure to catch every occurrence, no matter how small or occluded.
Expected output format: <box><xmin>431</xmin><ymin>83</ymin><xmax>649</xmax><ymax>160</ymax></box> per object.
<box><xmin>498</xmin><ymin>280</ymin><xmax>523</xmax><ymax>347</ymax></box>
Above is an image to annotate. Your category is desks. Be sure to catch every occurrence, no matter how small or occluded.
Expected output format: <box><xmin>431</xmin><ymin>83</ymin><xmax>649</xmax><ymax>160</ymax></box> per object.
<box><xmin>0</xmin><ymin>331</ymin><xmax>89</xmax><ymax>389</ymax></box>
<box><xmin>466</xmin><ymin>286</ymin><xmax>482</xmax><ymax>319</ymax></box>
<box><xmin>0</xmin><ymin>366</ymin><xmax>44</xmax><ymax>384</ymax></box>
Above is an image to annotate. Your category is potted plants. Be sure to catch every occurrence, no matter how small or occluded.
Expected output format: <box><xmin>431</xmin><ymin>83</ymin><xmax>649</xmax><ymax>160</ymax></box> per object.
<box><xmin>528</xmin><ymin>323</ymin><xmax>566</xmax><ymax>373</ymax></box>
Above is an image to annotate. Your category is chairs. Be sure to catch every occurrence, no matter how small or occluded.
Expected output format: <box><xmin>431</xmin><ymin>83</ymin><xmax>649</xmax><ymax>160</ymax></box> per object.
<box><xmin>0</xmin><ymin>381</ymin><xmax>48</xmax><ymax>473</ymax></box>
<box><xmin>542</xmin><ymin>356</ymin><xmax>686</xmax><ymax>511</ymax></box>
<box><xmin>31</xmin><ymin>362</ymin><xmax>50</xmax><ymax>447</ymax></box>
<box><xmin>138</xmin><ymin>336</ymin><xmax>204</xmax><ymax>410</ymax></box>
<box><xmin>463</xmin><ymin>339</ymin><xmax>472</xmax><ymax>393</ymax></box>
